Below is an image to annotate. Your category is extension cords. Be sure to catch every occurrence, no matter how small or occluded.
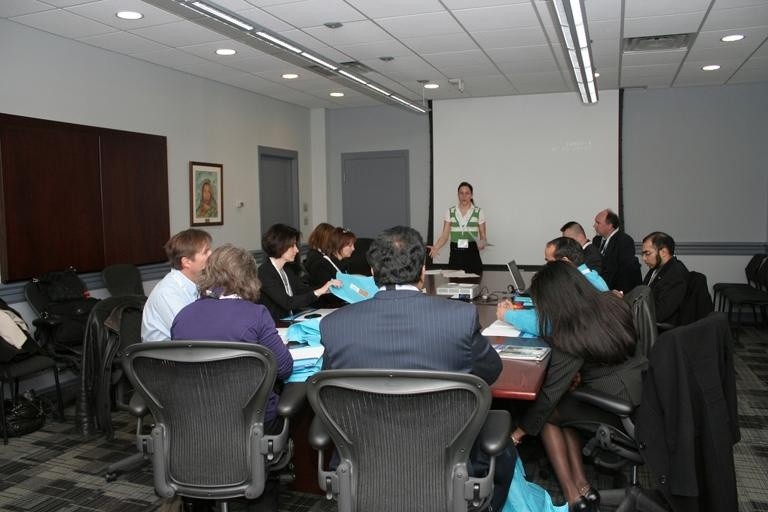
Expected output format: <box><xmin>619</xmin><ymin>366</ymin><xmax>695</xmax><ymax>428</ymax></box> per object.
<box><xmin>481</xmin><ymin>293</ymin><xmax>489</xmax><ymax>299</ymax></box>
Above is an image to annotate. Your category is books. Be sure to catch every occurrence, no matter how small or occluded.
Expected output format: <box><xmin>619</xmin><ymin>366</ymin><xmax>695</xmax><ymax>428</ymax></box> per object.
<box><xmin>480</xmin><ymin>319</ymin><xmax>538</xmax><ymax>338</ymax></box>
<box><xmin>490</xmin><ymin>343</ymin><xmax>552</xmax><ymax>361</ymax></box>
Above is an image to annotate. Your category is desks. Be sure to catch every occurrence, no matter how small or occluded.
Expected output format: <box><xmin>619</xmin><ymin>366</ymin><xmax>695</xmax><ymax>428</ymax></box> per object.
<box><xmin>272</xmin><ymin>269</ymin><xmax>554</xmax><ymax>401</ymax></box>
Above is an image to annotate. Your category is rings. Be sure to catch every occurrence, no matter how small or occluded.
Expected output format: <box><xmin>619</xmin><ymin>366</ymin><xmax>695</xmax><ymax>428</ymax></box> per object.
<box><xmin>331</xmin><ymin>279</ymin><xmax>333</xmax><ymax>281</ymax></box>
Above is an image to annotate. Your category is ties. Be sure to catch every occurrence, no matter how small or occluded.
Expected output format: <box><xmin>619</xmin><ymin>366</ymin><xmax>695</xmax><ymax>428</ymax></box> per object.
<box><xmin>599</xmin><ymin>240</ymin><xmax>606</xmax><ymax>254</ymax></box>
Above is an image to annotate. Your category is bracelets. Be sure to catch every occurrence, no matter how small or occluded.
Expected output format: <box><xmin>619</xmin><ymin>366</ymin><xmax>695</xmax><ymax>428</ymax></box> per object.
<box><xmin>511</xmin><ymin>436</ymin><xmax>522</xmax><ymax>443</ymax></box>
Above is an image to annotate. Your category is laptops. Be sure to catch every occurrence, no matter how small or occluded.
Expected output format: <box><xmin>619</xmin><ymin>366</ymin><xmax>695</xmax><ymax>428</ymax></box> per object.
<box><xmin>507</xmin><ymin>260</ymin><xmax>530</xmax><ymax>295</ymax></box>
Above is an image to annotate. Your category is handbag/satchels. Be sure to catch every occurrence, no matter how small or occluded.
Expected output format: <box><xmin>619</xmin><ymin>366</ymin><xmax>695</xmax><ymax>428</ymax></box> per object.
<box><xmin>32</xmin><ymin>267</ymin><xmax>90</xmax><ymax>303</ymax></box>
<box><xmin>41</xmin><ymin>299</ymin><xmax>105</xmax><ymax>340</ymax></box>
<box><xmin>0</xmin><ymin>393</ymin><xmax>49</xmax><ymax>438</ymax></box>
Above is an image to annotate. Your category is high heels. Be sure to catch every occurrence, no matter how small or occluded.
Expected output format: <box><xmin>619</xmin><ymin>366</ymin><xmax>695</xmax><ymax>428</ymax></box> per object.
<box><xmin>570</xmin><ymin>495</ymin><xmax>590</xmax><ymax>512</ymax></box>
<box><xmin>584</xmin><ymin>487</ymin><xmax>601</xmax><ymax>512</ymax></box>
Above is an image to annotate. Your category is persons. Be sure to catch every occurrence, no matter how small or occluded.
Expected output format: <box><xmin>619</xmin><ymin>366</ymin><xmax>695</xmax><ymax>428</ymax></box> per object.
<box><xmin>307</xmin><ymin>223</ymin><xmax>335</xmax><ymax>273</ymax></box>
<box><xmin>561</xmin><ymin>222</ymin><xmax>609</xmax><ymax>288</ymax></box>
<box><xmin>497</xmin><ymin>236</ymin><xmax>610</xmax><ymax>337</ymax></box>
<box><xmin>310</xmin><ymin>227</ymin><xmax>356</xmax><ymax>309</ymax></box>
<box><xmin>612</xmin><ymin>231</ymin><xmax>698</xmax><ymax>334</ymax></box>
<box><xmin>320</xmin><ymin>226</ymin><xmax>503</xmax><ymax>477</ymax></box>
<box><xmin>426</xmin><ymin>181</ymin><xmax>486</xmax><ymax>284</ymax></box>
<box><xmin>170</xmin><ymin>244</ymin><xmax>299</xmax><ymax>485</ymax></box>
<box><xmin>196</xmin><ymin>179</ymin><xmax>218</xmax><ymax>218</ymax></box>
<box><xmin>140</xmin><ymin>230</ymin><xmax>213</xmax><ymax>343</ymax></box>
<box><xmin>255</xmin><ymin>224</ymin><xmax>343</xmax><ymax>324</ymax></box>
<box><xmin>509</xmin><ymin>259</ymin><xmax>650</xmax><ymax>512</ymax></box>
<box><xmin>592</xmin><ymin>209</ymin><xmax>636</xmax><ymax>284</ymax></box>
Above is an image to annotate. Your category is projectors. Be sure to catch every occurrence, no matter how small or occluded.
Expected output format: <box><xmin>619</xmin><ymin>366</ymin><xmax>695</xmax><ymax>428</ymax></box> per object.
<box><xmin>437</xmin><ymin>282</ymin><xmax>480</xmax><ymax>300</ymax></box>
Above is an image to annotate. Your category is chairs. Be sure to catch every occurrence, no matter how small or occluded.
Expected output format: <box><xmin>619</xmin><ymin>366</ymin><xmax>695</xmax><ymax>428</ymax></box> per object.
<box><xmin>119</xmin><ymin>341</ymin><xmax>294</xmax><ymax>512</ymax></box>
<box><xmin>564</xmin><ymin>311</ymin><xmax>737</xmax><ymax>512</ymax></box>
<box><xmin>0</xmin><ymin>299</ymin><xmax>66</xmax><ymax>444</ymax></box>
<box><xmin>305</xmin><ymin>368</ymin><xmax>512</xmax><ymax>512</ymax></box>
<box><xmin>710</xmin><ymin>254</ymin><xmax>762</xmax><ymax>312</ymax></box>
<box><xmin>88</xmin><ymin>296</ymin><xmax>143</xmax><ymax>485</ymax></box>
<box><xmin>101</xmin><ymin>260</ymin><xmax>145</xmax><ymax>299</ymax></box>
<box><xmin>27</xmin><ymin>278</ymin><xmax>92</xmax><ymax>360</ymax></box>
<box><xmin>720</xmin><ymin>261</ymin><xmax>768</xmax><ymax>322</ymax></box>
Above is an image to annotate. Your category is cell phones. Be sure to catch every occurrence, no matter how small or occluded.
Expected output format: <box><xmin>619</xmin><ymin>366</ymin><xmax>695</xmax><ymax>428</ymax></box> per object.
<box><xmin>304</xmin><ymin>314</ymin><xmax>321</xmax><ymax>318</ymax></box>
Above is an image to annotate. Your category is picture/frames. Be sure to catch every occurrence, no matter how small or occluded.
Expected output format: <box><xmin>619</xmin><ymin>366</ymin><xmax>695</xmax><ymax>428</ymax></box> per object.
<box><xmin>189</xmin><ymin>161</ymin><xmax>225</xmax><ymax>227</ymax></box>
